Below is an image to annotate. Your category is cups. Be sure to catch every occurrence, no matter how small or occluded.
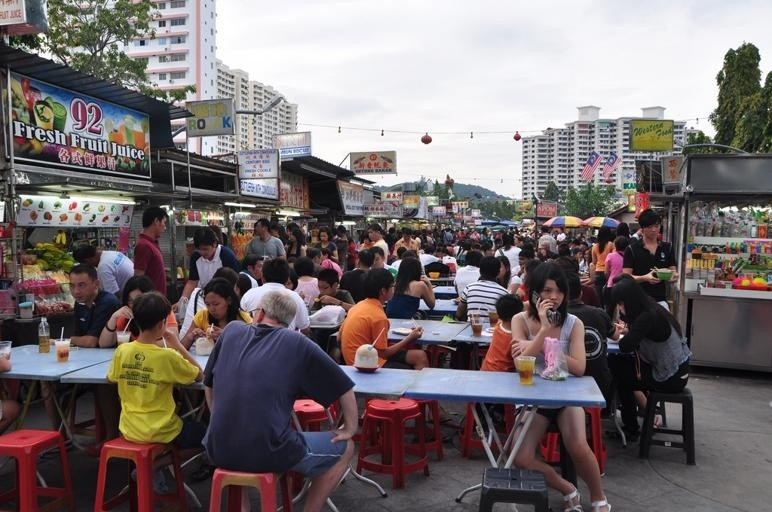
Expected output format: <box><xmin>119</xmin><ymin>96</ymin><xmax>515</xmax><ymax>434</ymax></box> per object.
<box><xmin>117</xmin><ymin>331</ymin><xmax>131</xmax><ymax>346</ymax></box>
<box><xmin>55</xmin><ymin>339</ymin><xmax>72</xmax><ymax>361</ymax></box>
<box><xmin>0</xmin><ymin>341</ymin><xmax>12</xmax><ymax>372</ymax></box>
<box><xmin>516</xmin><ymin>355</ymin><xmax>536</xmax><ymax>385</ymax></box>
<box><xmin>487</xmin><ymin>306</ymin><xmax>498</xmax><ymax>327</ymax></box>
<box><xmin>470</xmin><ymin>313</ymin><xmax>483</xmax><ymax>337</ymax></box>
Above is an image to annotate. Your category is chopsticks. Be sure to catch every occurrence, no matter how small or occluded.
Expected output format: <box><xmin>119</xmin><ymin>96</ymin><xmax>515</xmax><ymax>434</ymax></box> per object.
<box><xmin>410</xmin><ymin>317</ymin><xmax>424</xmax><ymax>334</ymax></box>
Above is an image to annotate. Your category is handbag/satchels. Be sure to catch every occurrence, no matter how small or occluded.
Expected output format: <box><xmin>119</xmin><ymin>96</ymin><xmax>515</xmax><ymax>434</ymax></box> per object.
<box><xmin>443</xmin><ymin>256</ymin><xmax>456</xmax><ymax>273</ymax></box>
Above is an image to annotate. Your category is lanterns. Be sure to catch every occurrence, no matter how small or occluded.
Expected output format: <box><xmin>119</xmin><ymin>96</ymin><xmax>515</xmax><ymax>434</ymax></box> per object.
<box><xmin>420</xmin><ymin>132</ymin><xmax>432</xmax><ymax>145</ymax></box>
<box><xmin>514</xmin><ymin>132</ymin><xmax>522</xmax><ymax>142</ymax></box>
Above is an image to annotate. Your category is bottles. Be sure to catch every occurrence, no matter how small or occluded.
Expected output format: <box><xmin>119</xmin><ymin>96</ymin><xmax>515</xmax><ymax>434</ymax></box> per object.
<box><xmin>38</xmin><ymin>317</ymin><xmax>51</xmax><ymax>353</ymax></box>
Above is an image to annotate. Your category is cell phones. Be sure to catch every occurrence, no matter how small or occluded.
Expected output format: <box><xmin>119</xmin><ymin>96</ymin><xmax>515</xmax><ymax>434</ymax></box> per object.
<box><xmin>531</xmin><ymin>290</ymin><xmax>561</xmax><ymax>326</ymax></box>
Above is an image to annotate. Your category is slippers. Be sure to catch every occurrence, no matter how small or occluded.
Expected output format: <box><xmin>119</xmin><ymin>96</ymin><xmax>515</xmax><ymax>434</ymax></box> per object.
<box><xmin>425</xmin><ymin>429</ymin><xmax>451</xmax><ymax>443</ymax></box>
<box><xmin>427</xmin><ymin>414</ymin><xmax>462</xmax><ymax>428</ymax></box>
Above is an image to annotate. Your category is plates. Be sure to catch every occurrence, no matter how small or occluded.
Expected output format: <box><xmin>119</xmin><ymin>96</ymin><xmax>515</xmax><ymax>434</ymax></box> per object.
<box><xmin>355</xmin><ymin>366</ymin><xmax>378</xmax><ymax>372</ymax></box>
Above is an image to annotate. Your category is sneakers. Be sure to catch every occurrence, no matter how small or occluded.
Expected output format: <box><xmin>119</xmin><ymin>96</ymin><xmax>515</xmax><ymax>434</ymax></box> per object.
<box><xmin>132</xmin><ymin>469</ymin><xmax>168</xmax><ymax>496</ymax></box>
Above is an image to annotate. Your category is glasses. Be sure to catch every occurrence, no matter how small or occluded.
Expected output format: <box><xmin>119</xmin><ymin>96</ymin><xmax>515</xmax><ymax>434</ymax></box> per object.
<box><xmin>316</xmin><ymin>285</ymin><xmax>330</xmax><ymax>291</ymax></box>
<box><xmin>193</xmin><ymin>246</ymin><xmax>212</xmax><ymax>254</ymax></box>
<box><xmin>390</xmin><ymin>284</ymin><xmax>397</xmax><ymax>288</ymax></box>
<box><xmin>250</xmin><ymin>307</ymin><xmax>264</xmax><ymax>317</ymax></box>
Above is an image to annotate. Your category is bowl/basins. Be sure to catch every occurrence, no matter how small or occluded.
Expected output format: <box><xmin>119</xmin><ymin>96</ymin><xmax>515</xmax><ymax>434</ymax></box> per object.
<box><xmin>655</xmin><ymin>271</ymin><xmax>672</xmax><ymax>281</ymax></box>
<box><xmin>429</xmin><ymin>272</ymin><xmax>440</xmax><ymax>279</ymax></box>
<box><xmin>196</xmin><ymin>338</ymin><xmax>214</xmax><ymax>355</ymax></box>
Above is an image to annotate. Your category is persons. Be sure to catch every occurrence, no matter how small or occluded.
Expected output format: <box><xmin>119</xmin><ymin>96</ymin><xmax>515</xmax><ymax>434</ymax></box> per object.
<box><xmin>105</xmin><ymin>289</ymin><xmax>205</xmax><ymax>495</ymax></box>
<box><xmin>510</xmin><ymin>261</ymin><xmax>611</xmax><ymax>512</ymax></box>
<box><xmin>199</xmin><ymin>286</ymin><xmax>359</xmax><ymax>511</ymax></box>
<box><xmin>0</xmin><ymin>206</ymin><xmax>692</xmax><ymax>474</ymax></box>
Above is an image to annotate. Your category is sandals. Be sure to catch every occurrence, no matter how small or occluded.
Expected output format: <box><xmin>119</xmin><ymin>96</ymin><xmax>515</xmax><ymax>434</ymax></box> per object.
<box><xmin>592</xmin><ymin>496</ymin><xmax>612</xmax><ymax>512</ymax></box>
<box><xmin>564</xmin><ymin>488</ymin><xmax>583</xmax><ymax>512</ymax></box>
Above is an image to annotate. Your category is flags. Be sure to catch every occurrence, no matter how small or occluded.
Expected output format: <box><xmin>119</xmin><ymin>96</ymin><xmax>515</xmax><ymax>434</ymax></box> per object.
<box><xmin>579</xmin><ymin>151</ymin><xmax>603</xmax><ymax>183</ymax></box>
<box><xmin>601</xmin><ymin>150</ymin><xmax>625</xmax><ymax>182</ymax></box>
